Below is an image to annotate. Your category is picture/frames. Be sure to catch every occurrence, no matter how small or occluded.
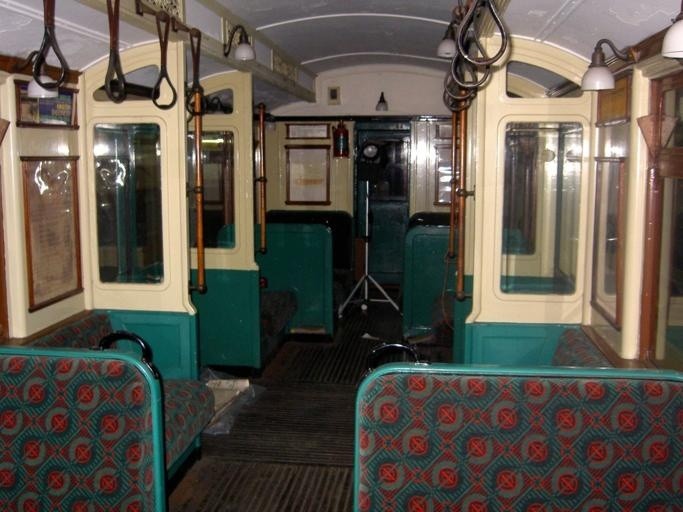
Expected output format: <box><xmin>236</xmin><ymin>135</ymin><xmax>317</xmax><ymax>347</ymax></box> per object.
<box><xmin>20</xmin><ymin>156</ymin><xmax>83</xmax><ymax>314</ymax></box>
<box><xmin>284</xmin><ymin>122</ymin><xmax>330</xmax><ymax>138</ymax></box>
<box><xmin>283</xmin><ymin>145</ymin><xmax>332</xmax><ymax>206</ymax></box>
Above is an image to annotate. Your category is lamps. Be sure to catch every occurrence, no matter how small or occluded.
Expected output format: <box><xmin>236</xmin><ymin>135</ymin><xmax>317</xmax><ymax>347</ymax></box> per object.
<box><xmin>437</xmin><ymin>21</ymin><xmax>457</xmax><ymax>60</ymax></box>
<box><xmin>222</xmin><ymin>23</ymin><xmax>255</xmax><ymax>62</ymax></box>
<box><xmin>375</xmin><ymin>91</ymin><xmax>388</xmax><ymax>111</ymax></box>
<box><xmin>581</xmin><ymin>39</ymin><xmax>646</xmax><ymax>92</ymax></box>
<box><xmin>657</xmin><ymin>1</ymin><xmax>683</xmax><ymax>60</ymax></box>
<box><xmin>14</xmin><ymin>51</ymin><xmax>59</xmax><ymax>99</ymax></box>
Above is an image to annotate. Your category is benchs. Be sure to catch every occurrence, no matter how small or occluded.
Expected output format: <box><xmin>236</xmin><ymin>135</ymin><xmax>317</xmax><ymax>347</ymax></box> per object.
<box><xmin>2</xmin><ymin>310</ymin><xmax>215</xmax><ymax>487</ymax></box>
<box><xmin>550</xmin><ymin>329</ymin><xmax>625</xmax><ymax>374</ymax></box>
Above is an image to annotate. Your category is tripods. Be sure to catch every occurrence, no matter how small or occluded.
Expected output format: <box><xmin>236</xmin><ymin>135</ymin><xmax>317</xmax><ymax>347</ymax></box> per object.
<box><xmin>338</xmin><ymin>183</ymin><xmax>403</xmax><ymax>319</ymax></box>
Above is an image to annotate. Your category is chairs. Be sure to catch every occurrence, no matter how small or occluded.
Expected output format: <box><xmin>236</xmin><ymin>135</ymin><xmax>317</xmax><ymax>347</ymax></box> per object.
<box><xmin>216</xmin><ymin>290</ymin><xmax>296</xmax><ymax>377</ymax></box>
<box><xmin>403</xmin><ymin>212</ymin><xmax>528</xmax><ymax>364</ymax></box>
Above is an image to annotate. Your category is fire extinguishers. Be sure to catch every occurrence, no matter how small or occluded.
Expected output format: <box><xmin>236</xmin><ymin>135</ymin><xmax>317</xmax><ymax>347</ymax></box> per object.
<box><xmin>332</xmin><ymin>119</ymin><xmax>349</xmax><ymax>158</ymax></box>
